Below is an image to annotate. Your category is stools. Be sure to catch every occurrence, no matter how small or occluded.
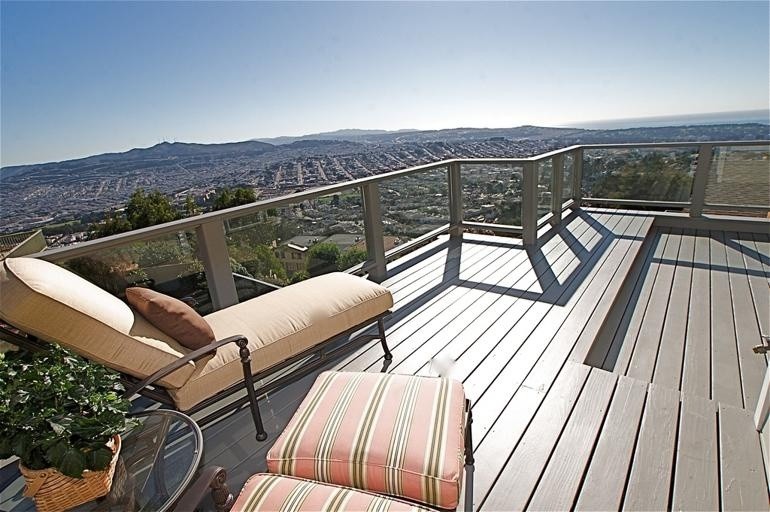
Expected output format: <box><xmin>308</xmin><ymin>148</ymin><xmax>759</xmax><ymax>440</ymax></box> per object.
<box><xmin>266</xmin><ymin>371</ymin><xmax>475</xmax><ymax>509</ymax></box>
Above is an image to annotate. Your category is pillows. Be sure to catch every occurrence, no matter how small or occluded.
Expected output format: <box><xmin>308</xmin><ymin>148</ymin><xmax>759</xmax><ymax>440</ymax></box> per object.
<box><xmin>124</xmin><ymin>286</ymin><xmax>217</xmax><ymax>355</ymax></box>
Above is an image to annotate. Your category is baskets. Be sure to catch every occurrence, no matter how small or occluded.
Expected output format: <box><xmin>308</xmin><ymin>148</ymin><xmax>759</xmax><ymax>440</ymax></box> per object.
<box><xmin>18</xmin><ymin>432</ymin><xmax>122</xmax><ymax>512</ymax></box>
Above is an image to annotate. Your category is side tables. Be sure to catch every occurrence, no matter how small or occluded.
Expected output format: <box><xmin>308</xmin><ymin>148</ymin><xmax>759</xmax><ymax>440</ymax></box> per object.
<box><xmin>0</xmin><ymin>408</ymin><xmax>206</xmax><ymax>511</ymax></box>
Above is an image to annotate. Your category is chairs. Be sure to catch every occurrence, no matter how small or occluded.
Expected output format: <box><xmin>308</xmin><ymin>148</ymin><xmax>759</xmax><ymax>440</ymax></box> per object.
<box><xmin>1</xmin><ymin>257</ymin><xmax>394</xmax><ymax>441</ymax></box>
<box><xmin>174</xmin><ymin>466</ymin><xmax>440</xmax><ymax>512</ymax></box>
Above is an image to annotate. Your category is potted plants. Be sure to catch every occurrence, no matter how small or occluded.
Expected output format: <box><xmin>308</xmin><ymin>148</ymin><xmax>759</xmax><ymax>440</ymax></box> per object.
<box><xmin>0</xmin><ymin>342</ymin><xmax>143</xmax><ymax>511</ymax></box>
